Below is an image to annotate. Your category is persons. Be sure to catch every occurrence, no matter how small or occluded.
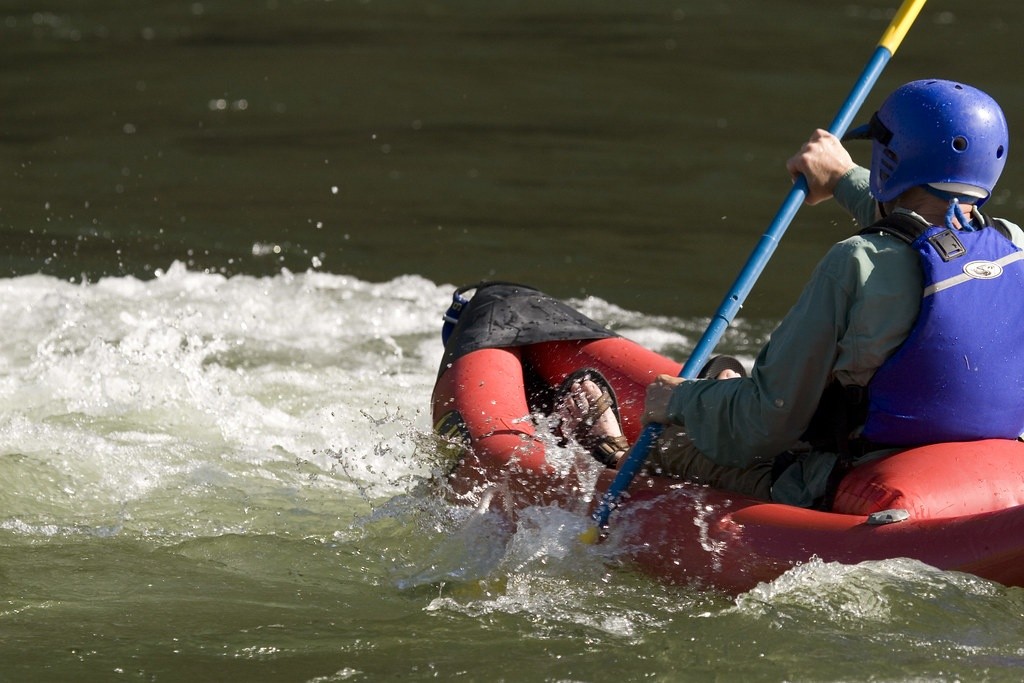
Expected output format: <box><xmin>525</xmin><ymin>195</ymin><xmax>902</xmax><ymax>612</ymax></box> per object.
<box><xmin>545</xmin><ymin>71</ymin><xmax>1024</xmax><ymax>548</ymax></box>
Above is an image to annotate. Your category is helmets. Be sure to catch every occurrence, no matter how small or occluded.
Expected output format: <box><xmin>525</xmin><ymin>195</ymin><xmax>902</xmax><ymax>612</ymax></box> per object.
<box><xmin>844</xmin><ymin>79</ymin><xmax>1008</xmax><ymax>210</ymax></box>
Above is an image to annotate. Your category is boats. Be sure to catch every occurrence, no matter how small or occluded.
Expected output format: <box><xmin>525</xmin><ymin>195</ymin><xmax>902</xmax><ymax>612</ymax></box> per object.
<box><xmin>428</xmin><ymin>278</ymin><xmax>1024</xmax><ymax>607</ymax></box>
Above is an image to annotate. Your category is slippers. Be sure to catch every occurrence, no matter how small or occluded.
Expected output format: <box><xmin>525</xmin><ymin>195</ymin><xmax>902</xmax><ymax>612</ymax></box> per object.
<box><xmin>554</xmin><ymin>367</ymin><xmax>628</xmax><ymax>468</ymax></box>
